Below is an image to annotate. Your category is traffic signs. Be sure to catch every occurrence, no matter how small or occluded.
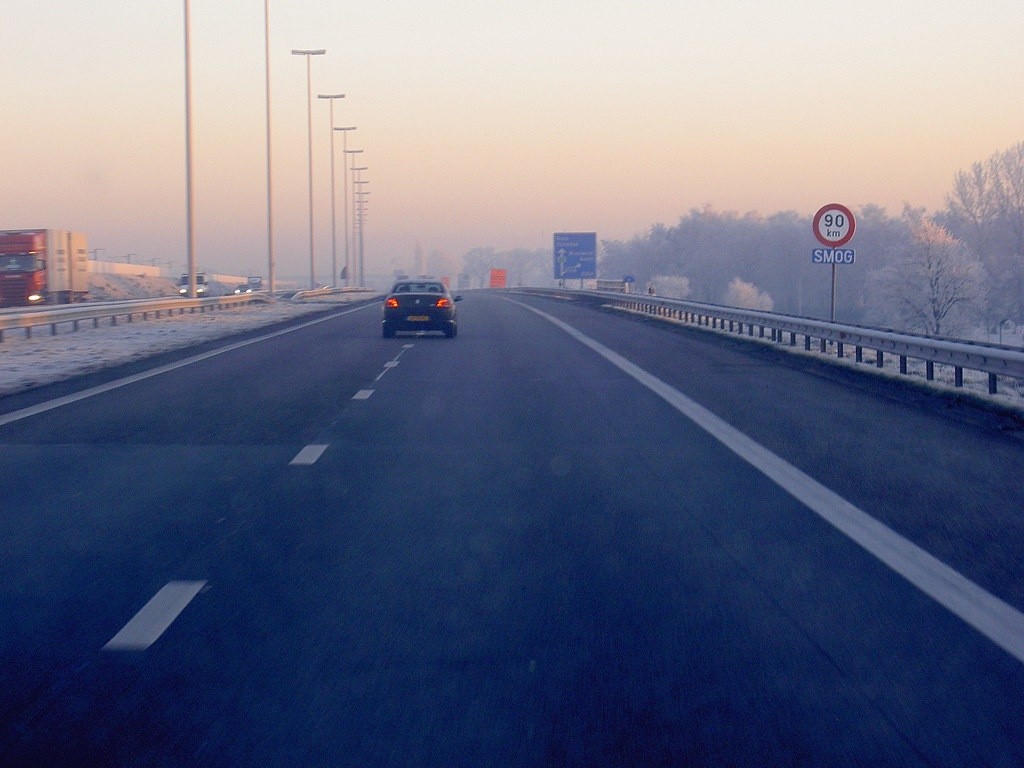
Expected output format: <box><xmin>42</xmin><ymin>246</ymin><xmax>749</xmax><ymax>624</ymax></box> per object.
<box><xmin>553</xmin><ymin>231</ymin><xmax>598</xmax><ymax>280</ymax></box>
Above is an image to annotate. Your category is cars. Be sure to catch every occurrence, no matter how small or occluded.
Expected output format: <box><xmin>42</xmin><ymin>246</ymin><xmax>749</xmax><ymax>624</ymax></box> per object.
<box><xmin>381</xmin><ymin>280</ymin><xmax>461</xmax><ymax>340</ymax></box>
<box><xmin>236</xmin><ymin>284</ymin><xmax>252</xmax><ymax>293</ymax></box>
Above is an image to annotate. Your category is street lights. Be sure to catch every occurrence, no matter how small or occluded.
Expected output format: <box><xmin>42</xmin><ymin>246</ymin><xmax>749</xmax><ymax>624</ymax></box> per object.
<box><xmin>332</xmin><ymin>125</ymin><xmax>359</xmax><ymax>287</ymax></box>
<box><xmin>315</xmin><ymin>94</ymin><xmax>349</xmax><ymax>290</ymax></box>
<box><xmin>342</xmin><ymin>148</ymin><xmax>372</xmax><ymax>288</ymax></box>
<box><xmin>291</xmin><ymin>49</ymin><xmax>327</xmax><ymax>290</ymax></box>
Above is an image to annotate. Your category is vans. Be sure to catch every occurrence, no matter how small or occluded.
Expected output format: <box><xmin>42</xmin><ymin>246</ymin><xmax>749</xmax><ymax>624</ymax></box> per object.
<box><xmin>179</xmin><ymin>272</ymin><xmax>209</xmax><ymax>298</ymax></box>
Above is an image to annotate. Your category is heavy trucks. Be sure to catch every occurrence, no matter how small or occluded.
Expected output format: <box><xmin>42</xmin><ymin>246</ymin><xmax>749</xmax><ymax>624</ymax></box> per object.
<box><xmin>0</xmin><ymin>230</ymin><xmax>88</xmax><ymax>306</ymax></box>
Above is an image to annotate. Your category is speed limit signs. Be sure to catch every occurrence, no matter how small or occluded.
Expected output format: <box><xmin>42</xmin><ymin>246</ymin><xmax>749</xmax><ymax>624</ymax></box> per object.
<box><xmin>812</xmin><ymin>203</ymin><xmax>856</xmax><ymax>249</ymax></box>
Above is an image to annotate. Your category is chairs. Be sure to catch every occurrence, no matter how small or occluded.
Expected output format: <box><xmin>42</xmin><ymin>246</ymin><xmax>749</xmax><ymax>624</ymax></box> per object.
<box><xmin>399</xmin><ymin>287</ymin><xmax>410</xmax><ymax>292</ymax></box>
<box><xmin>429</xmin><ymin>287</ymin><xmax>439</xmax><ymax>292</ymax></box>
